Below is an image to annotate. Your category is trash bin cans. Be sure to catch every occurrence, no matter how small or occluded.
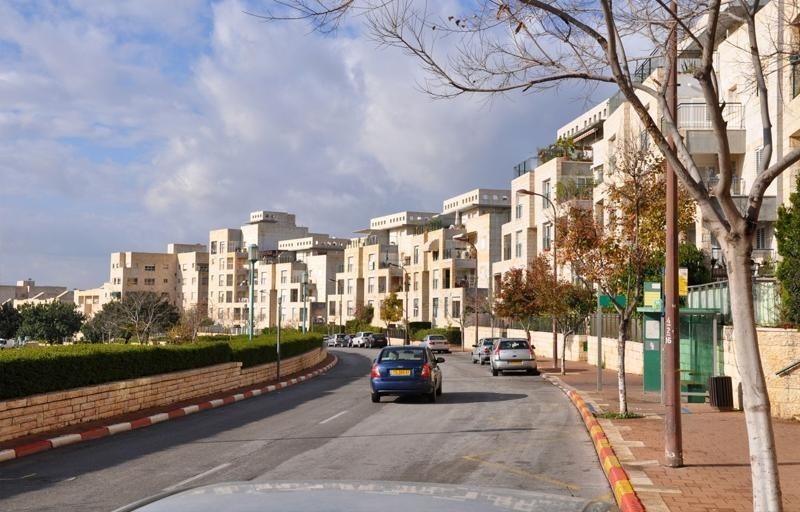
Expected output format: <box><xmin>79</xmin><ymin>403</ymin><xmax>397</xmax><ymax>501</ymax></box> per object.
<box><xmin>709</xmin><ymin>377</ymin><xmax>732</xmax><ymax>408</ymax></box>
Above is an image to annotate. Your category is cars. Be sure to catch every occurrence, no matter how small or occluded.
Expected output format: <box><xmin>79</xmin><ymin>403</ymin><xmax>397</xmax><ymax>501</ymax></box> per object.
<box><xmin>370</xmin><ymin>345</ymin><xmax>445</xmax><ymax>403</ymax></box>
<box><xmin>420</xmin><ymin>334</ymin><xmax>450</xmax><ymax>354</ymax></box>
<box><xmin>322</xmin><ymin>332</ymin><xmax>387</xmax><ymax>348</ymax></box>
<box><xmin>471</xmin><ymin>337</ymin><xmax>538</xmax><ymax>376</ymax></box>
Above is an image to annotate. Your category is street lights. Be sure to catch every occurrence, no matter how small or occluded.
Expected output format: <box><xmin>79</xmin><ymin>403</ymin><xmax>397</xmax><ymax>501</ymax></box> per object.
<box><xmin>248</xmin><ymin>244</ymin><xmax>259</xmax><ymax>341</ymax></box>
<box><xmin>301</xmin><ymin>271</ymin><xmax>308</xmax><ymax>333</ymax></box>
<box><xmin>453</xmin><ymin>234</ymin><xmax>479</xmax><ymax>346</ymax></box>
<box><xmin>328</xmin><ymin>278</ymin><xmax>342</xmax><ymax>333</ymax></box>
<box><xmin>516</xmin><ymin>188</ymin><xmax>558</xmax><ymax>369</ymax></box>
<box><xmin>388</xmin><ymin>262</ymin><xmax>410</xmax><ymax>345</ymax></box>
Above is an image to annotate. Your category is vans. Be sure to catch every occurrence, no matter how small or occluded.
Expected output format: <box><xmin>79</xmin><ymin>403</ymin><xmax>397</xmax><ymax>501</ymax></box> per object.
<box><xmin>0</xmin><ymin>338</ymin><xmax>15</xmax><ymax>347</ymax></box>
